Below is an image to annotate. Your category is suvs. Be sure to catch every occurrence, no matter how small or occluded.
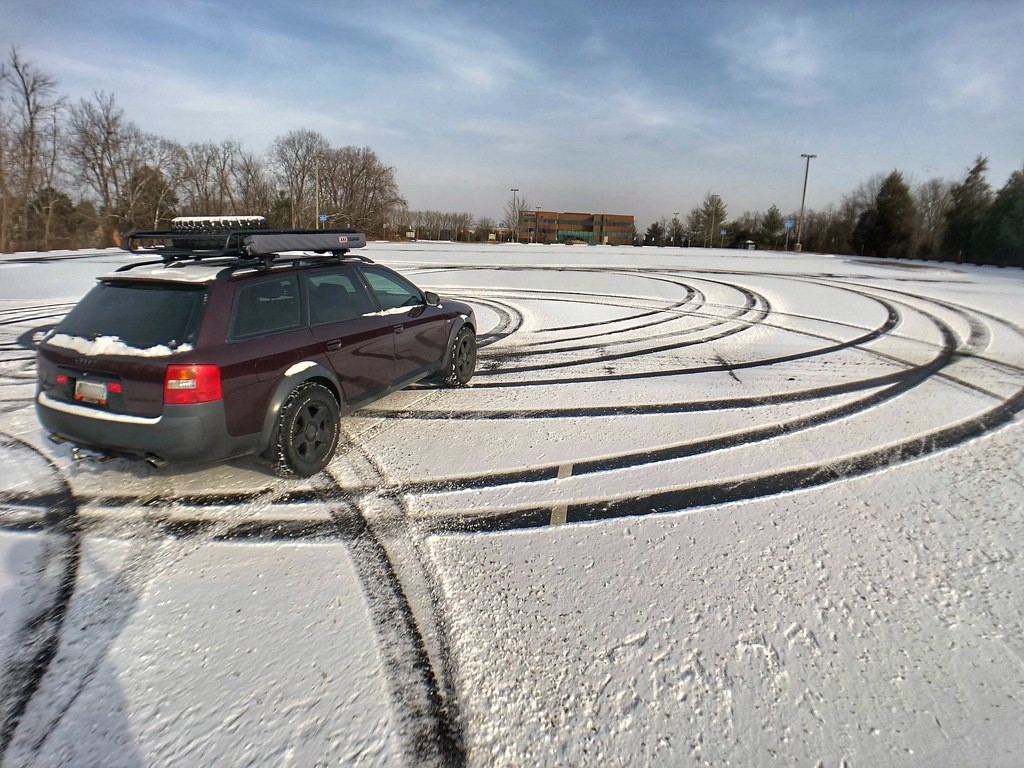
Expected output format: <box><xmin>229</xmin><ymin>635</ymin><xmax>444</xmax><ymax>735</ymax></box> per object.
<box><xmin>30</xmin><ymin>215</ymin><xmax>481</xmax><ymax>478</ymax></box>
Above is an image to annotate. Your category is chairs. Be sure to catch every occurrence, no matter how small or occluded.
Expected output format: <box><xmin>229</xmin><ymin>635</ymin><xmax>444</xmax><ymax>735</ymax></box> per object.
<box><xmin>316</xmin><ymin>283</ymin><xmax>348</xmax><ymax>319</ymax></box>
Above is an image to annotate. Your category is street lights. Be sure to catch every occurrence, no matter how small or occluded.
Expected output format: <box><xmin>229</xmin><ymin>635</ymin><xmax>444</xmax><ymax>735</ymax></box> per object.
<box><xmin>709</xmin><ymin>194</ymin><xmax>719</xmax><ymax>248</ymax></box>
<box><xmin>673</xmin><ymin>211</ymin><xmax>679</xmax><ymax>246</ymax></box>
<box><xmin>509</xmin><ymin>187</ymin><xmax>519</xmax><ymax>241</ymax></box>
<box><xmin>307</xmin><ymin>153</ymin><xmax>325</xmax><ymax>228</ymax></box>
<box><xmin>795</xmin><ymin>152</ymin><xmax>818</xmax><ymax>251</ymax></box>
<box><xmin>533</xmin><ymin>206</ymin><xmax>542</xmax><ymax>244</ymax></box>
